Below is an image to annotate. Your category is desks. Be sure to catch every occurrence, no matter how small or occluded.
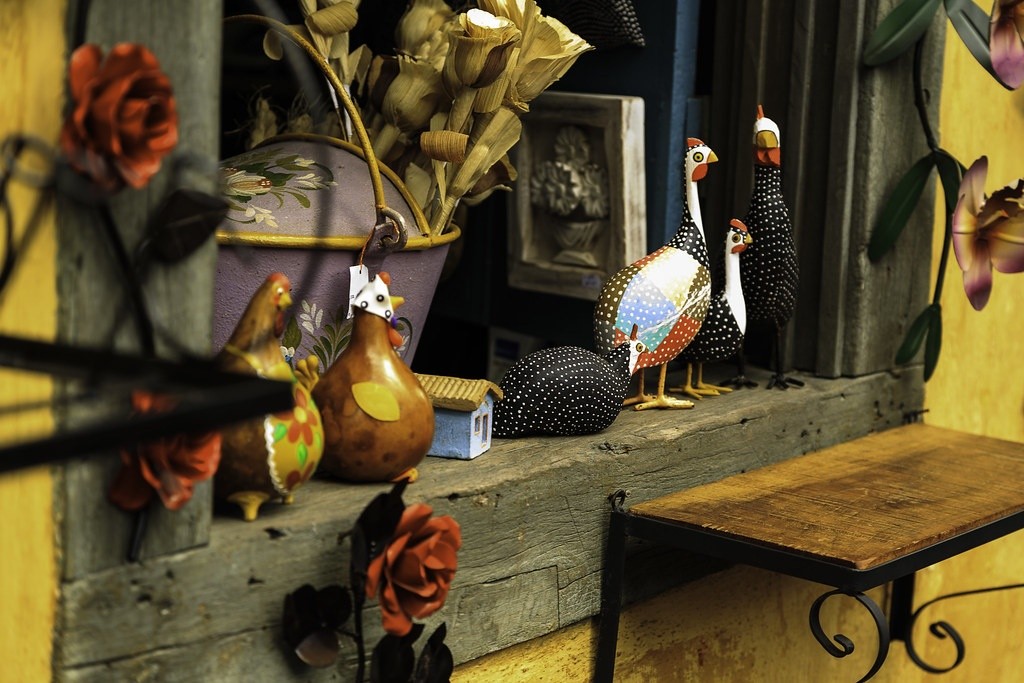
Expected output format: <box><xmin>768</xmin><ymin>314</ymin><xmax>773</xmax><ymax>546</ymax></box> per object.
<box><xmin>592</xmin><ymin>405</ymin><xmax>1023</xmax><ymax>682</ymax></box>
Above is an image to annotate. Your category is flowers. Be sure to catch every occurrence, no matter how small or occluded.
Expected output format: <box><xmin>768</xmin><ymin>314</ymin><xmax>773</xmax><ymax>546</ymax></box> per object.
<box><xmin>860</xmin><ymin>0</ymin><xmax>1024</xmax><ymax>385</ymax></box>
<box><xmin>57</xmin><ymin>0</ymin><xmax>594</xmax><ymax>682</ymax></box>
